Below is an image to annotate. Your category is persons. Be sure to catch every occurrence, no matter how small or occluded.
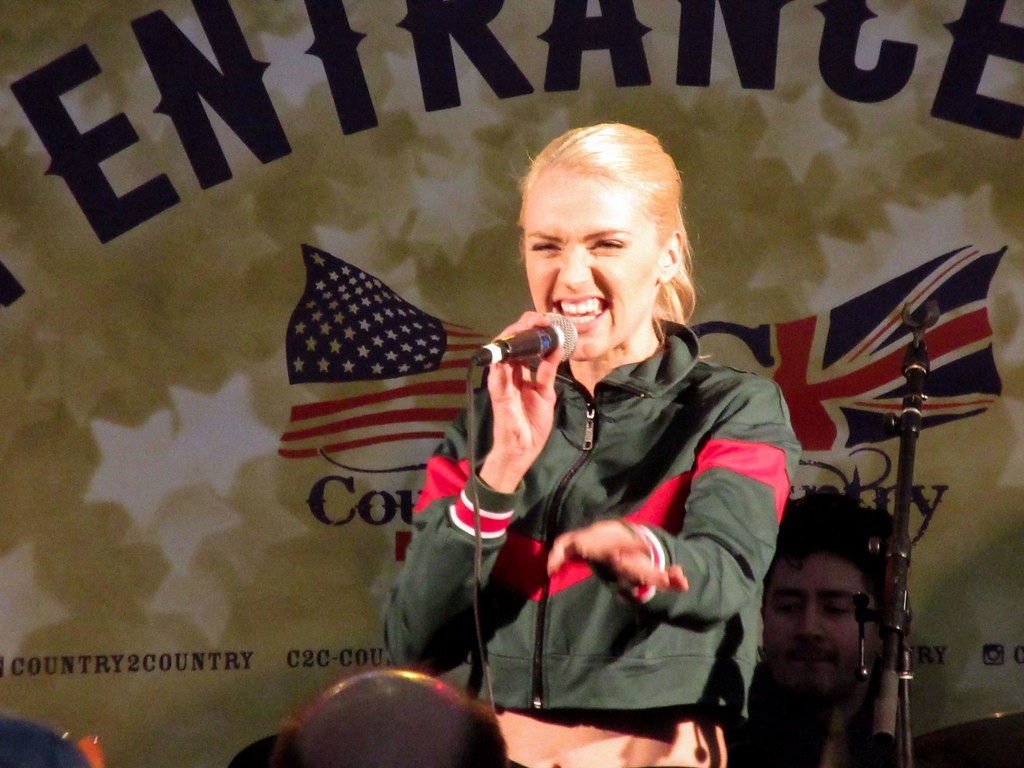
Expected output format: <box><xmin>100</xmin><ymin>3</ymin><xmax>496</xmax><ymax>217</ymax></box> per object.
<box><xmin>381</xmin><ymin>123</ymin><xmax>800</xmax><ymax>768</ymax></box>
<box><xmin>728</xmin><ymin>489</ymin><xmax>898</xmax><ymax>768</ymax></box>
<box><xmin>0</xmin><ymin>668</ymin><xmax>515</xmax><ymax>768</ymax></box>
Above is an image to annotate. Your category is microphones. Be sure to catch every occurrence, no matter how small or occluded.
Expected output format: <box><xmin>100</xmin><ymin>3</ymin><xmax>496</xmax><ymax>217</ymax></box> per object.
<box><xmin>473</xmin><ymin>312</ymin><xmax>578</xmax><ymax>366</ymax></box>
